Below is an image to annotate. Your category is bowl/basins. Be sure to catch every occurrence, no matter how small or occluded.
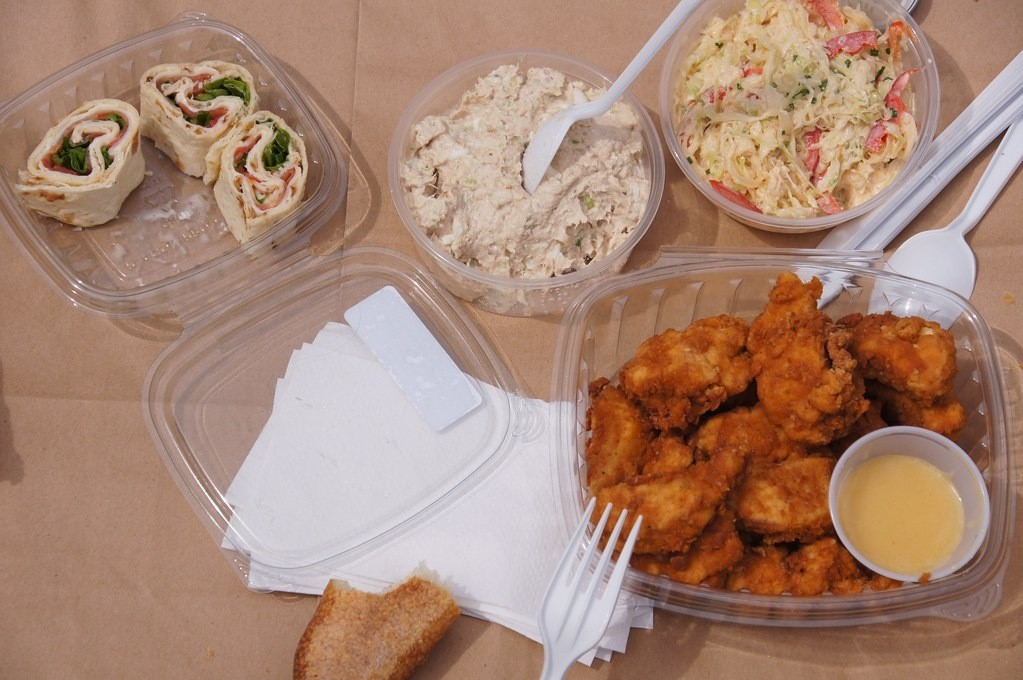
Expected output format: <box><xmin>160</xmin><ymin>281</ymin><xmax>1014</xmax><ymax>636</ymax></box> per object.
<box><xmin>546</xmin><ymin>253</ymin><xmax>1017</xmax><ymax>631</ymax></box>
<box><xmin>660</xmin><ymin>0</ymin><xmax>941</xmax><ymax>232</ymax></box>
<box><xmin>386</xmin><ymin>49</ymin><xmax>666</xmax><ymax>316</ymax></box>
<box><xmin>0</xmin><ymin>10</ymin><xmax>347</xmax><ymax>317</ymax></box>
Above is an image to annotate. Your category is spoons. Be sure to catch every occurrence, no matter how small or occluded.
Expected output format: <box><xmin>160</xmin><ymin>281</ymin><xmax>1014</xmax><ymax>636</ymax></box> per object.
<box><xmin>865</xmin><ymin>108</ymin><xmax>1023</xmax><ymax>332</ymax></box>
<box><xmin>519</xmin><ymin>0</ymin><xmax>707</xmax><ymax>197</ymax></box>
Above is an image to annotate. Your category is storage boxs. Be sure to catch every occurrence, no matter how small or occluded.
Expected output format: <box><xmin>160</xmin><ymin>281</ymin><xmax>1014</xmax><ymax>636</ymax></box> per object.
<box><xmin>0</xmin><ymin>0</ymin><xmax>1023</xmax><ymax>680</ymax></box>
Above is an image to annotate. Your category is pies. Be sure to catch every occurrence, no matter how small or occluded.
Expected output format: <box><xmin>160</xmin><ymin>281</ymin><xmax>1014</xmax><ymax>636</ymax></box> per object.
<box><xmin>14</xmin><ymin>59</ymin><xmax>308</xmax><ymax>257</ymax></box>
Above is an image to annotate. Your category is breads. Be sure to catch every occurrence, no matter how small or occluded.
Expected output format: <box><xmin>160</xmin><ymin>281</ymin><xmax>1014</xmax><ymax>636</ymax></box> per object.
<box><xmin>290</xmin><ymin>577</ymin><xmax>464</xmax><ymax>680</ymax></box>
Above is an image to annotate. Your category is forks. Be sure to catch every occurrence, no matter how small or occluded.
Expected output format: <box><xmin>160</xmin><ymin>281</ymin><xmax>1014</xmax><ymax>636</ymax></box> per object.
<box><xmin>537</xmin><ymin>494</ymin><xmax>643</xmax><ymax>680</ymax></box>
